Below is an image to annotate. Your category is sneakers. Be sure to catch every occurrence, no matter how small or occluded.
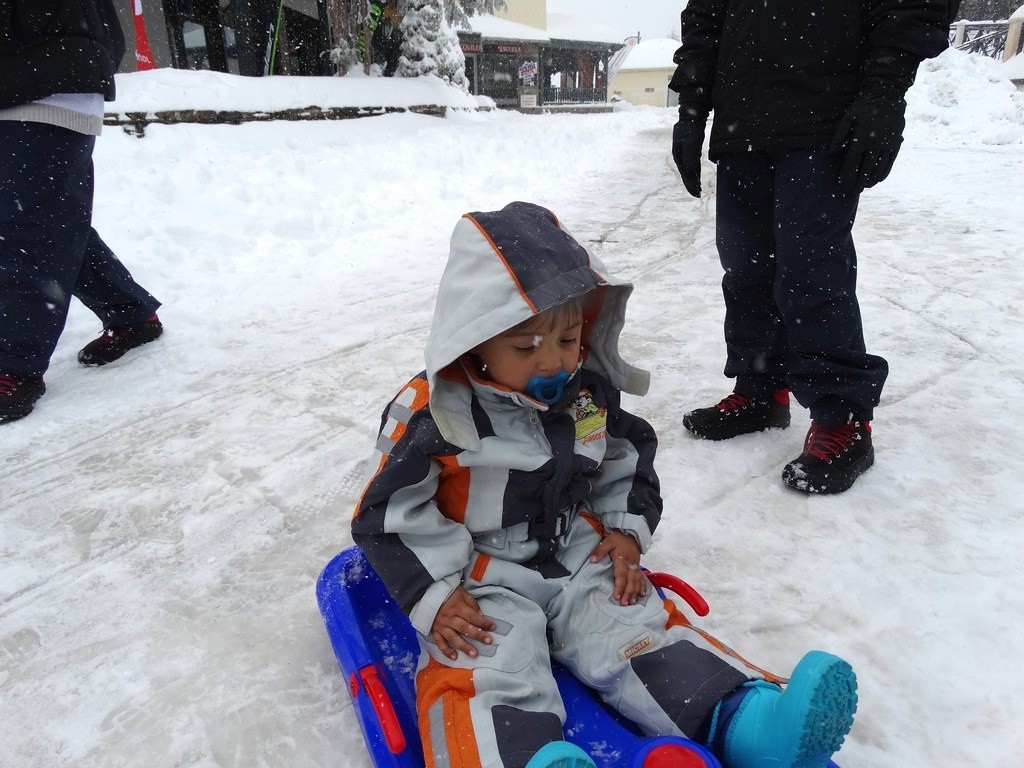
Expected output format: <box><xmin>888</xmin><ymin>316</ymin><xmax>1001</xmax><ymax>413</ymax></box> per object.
<box><xmin>0</xmin><ymin>360</ymin><xmax>48</xmax><ymax>422</ymax></box>
<box><xmin>687</xmin><ymin>373</ymin><xmax>793</xmax><ymax>442</ymax></box>
<box><xmin>80</xmin><ymin>308</ymin><xmax>162</xmax><ymax>365</ymax></box>
<box><xmin>780</xmin><ymin>402</ymin><xmax>879</xmax><ymax>496</ymax></box>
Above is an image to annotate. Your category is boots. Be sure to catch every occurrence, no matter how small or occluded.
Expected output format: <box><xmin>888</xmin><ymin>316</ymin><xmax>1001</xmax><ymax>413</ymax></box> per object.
<box><xmin>701</xmin><ymin>647</ymin><xmax>860</xmax><ymax>768</ymax></box>
<box><xmin>519</xmin><ymin>738</ymin><xmax>595</xmax><ymax>768</ymax></box>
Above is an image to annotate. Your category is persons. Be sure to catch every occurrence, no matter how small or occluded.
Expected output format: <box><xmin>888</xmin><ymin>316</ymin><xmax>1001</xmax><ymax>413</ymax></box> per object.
<box><xmin>0</xmin><ymin>4</ymin><xmax>169</xmax><ymax>426</ymax></box>
<box><xmin>351</xmin><ymin>202</ymin><xmax>859</xmax><ymax>767</ymax></box>
<box><xmin>667</xmin><ymin>3</ymin><xmax>961</xmax><ymax>497</ymax></box>
